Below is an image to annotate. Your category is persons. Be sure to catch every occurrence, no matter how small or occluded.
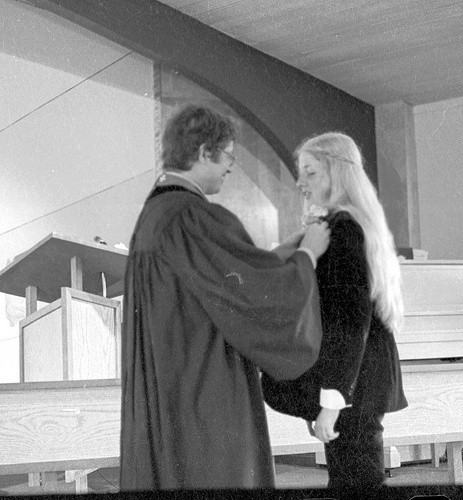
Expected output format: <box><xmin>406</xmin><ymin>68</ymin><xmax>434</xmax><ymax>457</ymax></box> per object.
<box><xmin>118</xmin><ymin>106</ymin><xmax>331</xmax><ymax>492</ymax></box>
<box><xmin>261</xmin><ymin>129</ymin><xmax>408</xmax><ymax>488</ymax></box>
